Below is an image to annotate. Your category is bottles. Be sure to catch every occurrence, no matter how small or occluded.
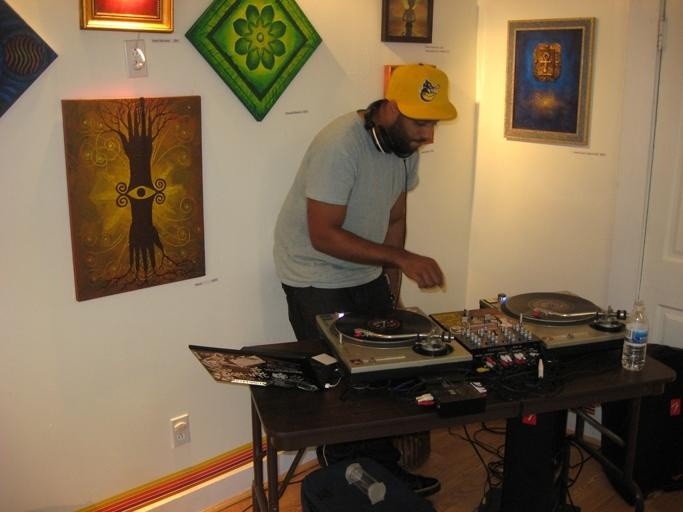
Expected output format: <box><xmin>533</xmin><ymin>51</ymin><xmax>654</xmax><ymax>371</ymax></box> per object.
<box><xmin>621</xmin><ymin>301</ymin><xmax>649</xmax><ymax>371</ymax></box>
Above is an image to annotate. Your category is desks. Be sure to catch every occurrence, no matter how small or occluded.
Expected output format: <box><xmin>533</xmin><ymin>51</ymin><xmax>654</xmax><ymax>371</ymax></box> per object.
<box><xmin>239</xmin><ymin>342</ymin><xmax>677</xmax><ymax>511</ymax></box>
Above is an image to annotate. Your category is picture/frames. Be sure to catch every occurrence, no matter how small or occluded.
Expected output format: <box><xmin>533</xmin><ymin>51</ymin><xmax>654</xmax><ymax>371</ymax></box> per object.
<box><xmin>380</xmin><ymin>0</ymin><xmax>434</xmax><ymax>44</ymax></box>
<box><xmin>501</xmin><ymin>15</ymin><xmax>596</xmax><ymax>148</ymax></box>
<box><xmin>77</xmin><ymin>0</ymin><xmax>175</xmax><ymax>34</ymax></box>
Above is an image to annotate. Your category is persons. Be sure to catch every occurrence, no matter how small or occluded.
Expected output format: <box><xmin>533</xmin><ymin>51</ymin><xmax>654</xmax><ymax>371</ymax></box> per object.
<box><xmin>269</xmin><ymin>61</ymin><xmax>459</xmax><ymax>500</ymax></box>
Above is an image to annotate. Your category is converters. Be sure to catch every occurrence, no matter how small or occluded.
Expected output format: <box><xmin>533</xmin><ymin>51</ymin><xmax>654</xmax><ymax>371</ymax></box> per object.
<box><xmin>431</xmin><ymin>384</ymin><xmax>487</xmax><ymax>418</ymax></box>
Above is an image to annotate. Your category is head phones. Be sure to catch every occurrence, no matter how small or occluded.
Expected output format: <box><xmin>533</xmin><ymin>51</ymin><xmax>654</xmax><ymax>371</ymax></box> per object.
<box><xmin>365</xmin><ymin>97</ymin><xmax>415</xmax><ymax>158</ymax></box>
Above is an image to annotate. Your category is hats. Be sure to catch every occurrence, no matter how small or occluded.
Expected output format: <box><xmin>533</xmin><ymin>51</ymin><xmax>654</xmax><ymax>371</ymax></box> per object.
<box><xmin>387</xmin><ymin>64</ymin><xmax>457</xmax><ymax>120</ymax></box>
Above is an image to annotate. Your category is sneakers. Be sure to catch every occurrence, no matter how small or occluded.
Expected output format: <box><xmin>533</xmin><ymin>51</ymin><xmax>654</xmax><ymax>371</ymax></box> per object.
<box><xmin>392</xmin><ymin>468</ymin><xmax>439</xmax><ymax>496</ymax></box>
<box><xmin>316</xmin><ymin>445</ymin><xmax>331</xmax><ymax>466</ymax></box>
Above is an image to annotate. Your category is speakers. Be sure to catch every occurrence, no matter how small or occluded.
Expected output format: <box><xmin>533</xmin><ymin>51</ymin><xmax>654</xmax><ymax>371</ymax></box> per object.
<box><xmin>301</xmin><ymin>455</ymin><xmax>435</xmax><ymax>511</ymax></box>
